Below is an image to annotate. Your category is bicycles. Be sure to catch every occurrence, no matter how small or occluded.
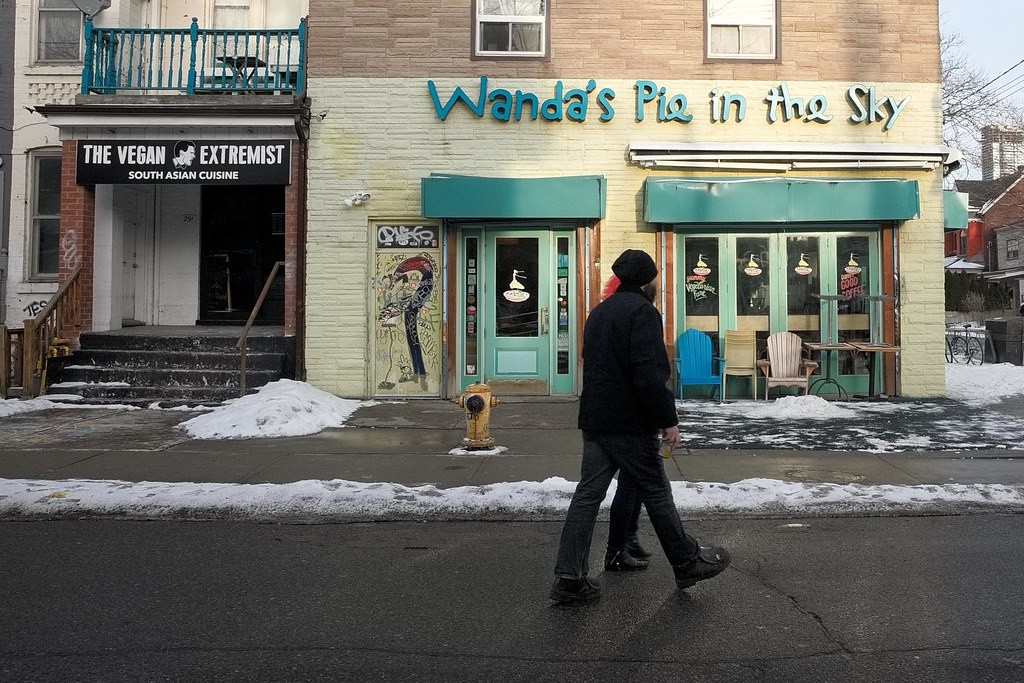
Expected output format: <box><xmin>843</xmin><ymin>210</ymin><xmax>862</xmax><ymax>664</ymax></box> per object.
<box><xmin>945</xmin><ymin>321</ymin><xmax>984</xmax><ymax>366</ymax></box>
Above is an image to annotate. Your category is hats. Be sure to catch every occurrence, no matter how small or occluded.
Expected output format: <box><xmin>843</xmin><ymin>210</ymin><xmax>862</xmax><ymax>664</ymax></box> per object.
<box><xmin>612</xmin><ymin>249</ymin><xmax>658</xmax><ymax>287</ymax></box>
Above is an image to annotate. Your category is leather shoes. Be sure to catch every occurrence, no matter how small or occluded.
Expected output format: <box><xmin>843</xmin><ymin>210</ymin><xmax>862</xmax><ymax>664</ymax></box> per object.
<box><xmin>604</xmin><ymin>550</ymin><xmax>649</xmax><ymax>572</ymax></box>
<box><xmin>624</xmin><ymin>536</ymin><xmax>654</xmax><ymax>558</ymax></box>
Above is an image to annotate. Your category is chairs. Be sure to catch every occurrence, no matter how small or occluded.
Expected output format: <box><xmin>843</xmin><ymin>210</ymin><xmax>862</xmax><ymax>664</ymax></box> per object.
<box><xmin>671</xmin><ymin>328</ymin><xmax>726</xmax><ymax>405</ymax></box>
<box><xmin>757</xmin><ymin>332</ymin><xmax>818</xmax><ymax>402</ymax></box>
<box><xmin>722</xmin><ymin>329</ymin><xmax>758</xmax><ymax>403</ymax></box>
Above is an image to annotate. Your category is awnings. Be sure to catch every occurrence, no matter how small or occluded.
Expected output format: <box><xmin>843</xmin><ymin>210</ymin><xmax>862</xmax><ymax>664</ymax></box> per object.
<box><xmin>420</xmin><ymin>170</ymin><xmax>606</xmax><ymax>219</ymax></box>
<box><xmin>945</xmin><ymin>256</ymin><xmax>985</xmax><ymax>274</ymax></box>
<box><xmin>643</xmin><ymin>176</ymin><xmax>921</xmax><ymax>228</ymax></box>
<box><xmin>943</xmin><ymin>191</ymin><xmax>969</xmax><ymax>234</ymax></box>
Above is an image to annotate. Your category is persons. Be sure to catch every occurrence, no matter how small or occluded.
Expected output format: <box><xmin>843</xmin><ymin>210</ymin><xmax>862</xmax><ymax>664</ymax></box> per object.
<box><xmin>548</xmin><ymin>249</ymin><xmax>731</xmax><ymax>603</ymax></box>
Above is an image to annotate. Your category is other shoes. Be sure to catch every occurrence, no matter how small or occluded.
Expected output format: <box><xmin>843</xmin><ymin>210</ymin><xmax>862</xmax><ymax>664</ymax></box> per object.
<box><xmin>673</xmin><ymin>547</ymin><xmax>732</xmax><ymax>589</ymax></box>
<box><xmin>548</xmin><ymin>575</ymin><xmax>602</xmax><ymax>601</ymax></box>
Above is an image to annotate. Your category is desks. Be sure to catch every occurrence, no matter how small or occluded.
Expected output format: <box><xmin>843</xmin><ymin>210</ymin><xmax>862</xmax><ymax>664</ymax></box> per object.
<box><xmin>215</xmin><ymin>55</ymin><xmax>267</xmax><ymax>95</ymax></box>
<box><xmin>848</xmin><ymin>342</ymin><xmax>904</xmax><ymax>402</ymax></box>
<box><xmin>804</xmin><ymin>340</ymin><xmax>856</xmax><ymax>402</ymax></box>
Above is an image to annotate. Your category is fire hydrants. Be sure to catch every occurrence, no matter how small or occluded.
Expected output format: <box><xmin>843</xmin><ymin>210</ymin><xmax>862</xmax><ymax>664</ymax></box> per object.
<box><xmin>451</xmin><ymin>381</ymin><xmax>501</xmax><ymax>450</ymax></box>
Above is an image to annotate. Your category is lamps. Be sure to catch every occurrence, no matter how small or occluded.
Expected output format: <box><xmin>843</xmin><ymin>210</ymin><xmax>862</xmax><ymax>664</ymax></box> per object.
<box><xmin>341</xmin><ymin>191</ymin><xmax>371</xmax><ymax>206</ymax></box>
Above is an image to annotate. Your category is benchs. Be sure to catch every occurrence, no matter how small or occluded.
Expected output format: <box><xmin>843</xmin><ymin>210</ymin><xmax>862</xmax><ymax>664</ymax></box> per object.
<box><xmin>270</xmin><ymin>63</ymin><xmax>298</xmax><ymax>95</ymax></box>
